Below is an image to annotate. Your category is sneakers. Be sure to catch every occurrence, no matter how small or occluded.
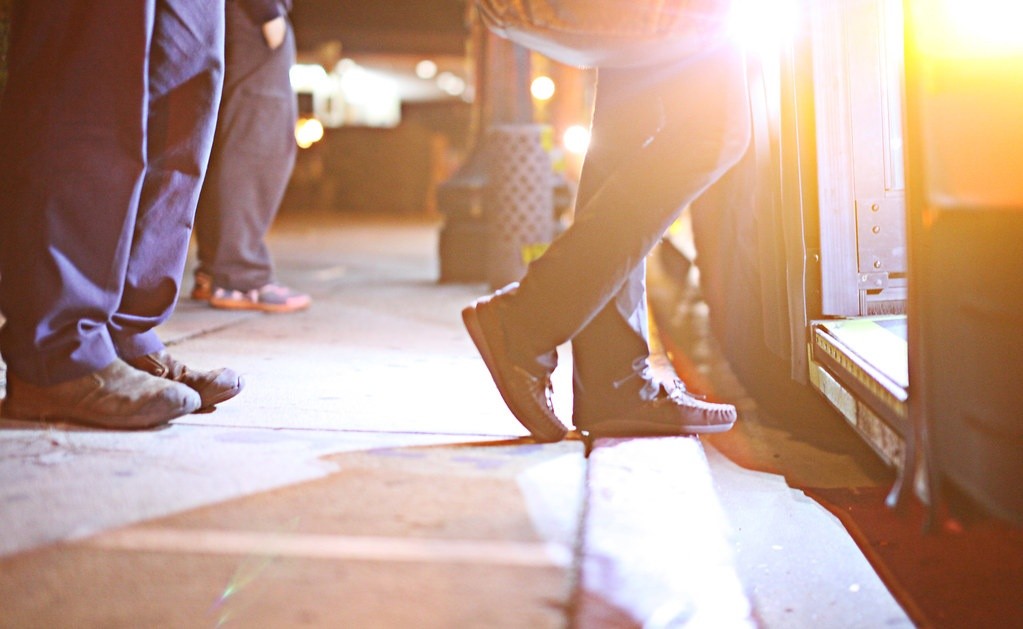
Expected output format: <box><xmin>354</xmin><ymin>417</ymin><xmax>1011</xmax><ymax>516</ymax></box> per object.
<box><xmin>463</xmin><ymin>291</ymin><xmax>567</xmax><ymax>441</ymax></box>
<box><xmin>576</xmin><ymin>380</ymin><xmax>737</xmax><ymax>434</ymax></box>
<box><xmin>2</xmin><ymin>355</ymin><xmax>201</xmax><ymax>427</ymax></box>
<box><xmin>127</xmin><ymin>352</ymin><xmax>244</xmax><ymax>406</ymax></box>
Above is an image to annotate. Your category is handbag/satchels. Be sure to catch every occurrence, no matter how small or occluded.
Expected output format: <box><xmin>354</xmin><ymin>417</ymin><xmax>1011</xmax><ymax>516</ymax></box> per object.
<box><xmin>476</xmin><ymin>0</ymin><xmax>727</xmax><ymax>68</ymax></box>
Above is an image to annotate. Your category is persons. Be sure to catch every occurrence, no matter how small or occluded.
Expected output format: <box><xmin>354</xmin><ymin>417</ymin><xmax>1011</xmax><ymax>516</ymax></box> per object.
<box><xmin>458</xmin><ymin>0</ymin><xmax>753</xmax><ymax>441</ymax></box>
<box><xmin>0</xmin><ymin>0</ymin><xmax>242</xmax><ymax>429</ymax></box>
<box><xmin>189</xmin><ymin>0</ymin><xmax>311</xmax><ymax>314</ymax></box>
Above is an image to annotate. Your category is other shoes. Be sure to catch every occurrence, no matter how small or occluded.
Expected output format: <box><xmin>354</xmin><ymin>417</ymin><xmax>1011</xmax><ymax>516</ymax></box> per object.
<box><xmin>209</xmin><ymin>280</ymin><xmax>310</xmax><ymax>313</ymax></box>
<box><xmin>190</xmin><ymin>267</ymin><xmax>213</xmax><ymax>299</ymax></box>
